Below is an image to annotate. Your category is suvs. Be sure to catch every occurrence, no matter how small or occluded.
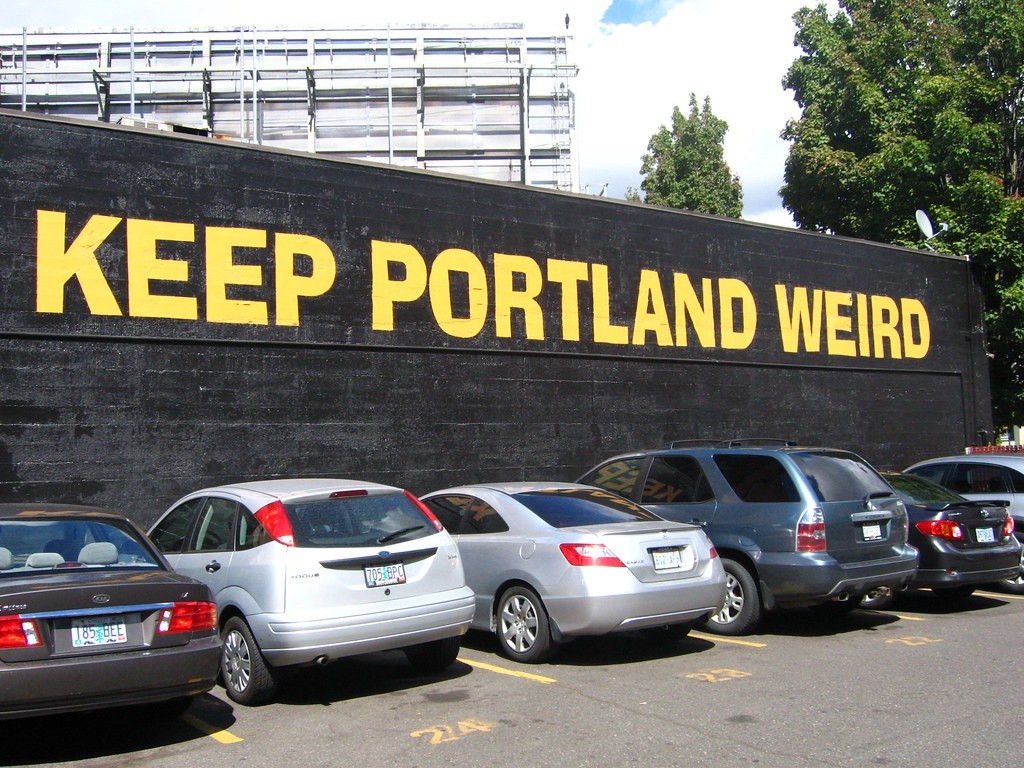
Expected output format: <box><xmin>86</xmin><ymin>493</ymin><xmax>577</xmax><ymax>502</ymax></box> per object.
<box><xmin>564</xmin><ymin>436</ymin><xmax>923</xmax><ymax>637</ymax></box>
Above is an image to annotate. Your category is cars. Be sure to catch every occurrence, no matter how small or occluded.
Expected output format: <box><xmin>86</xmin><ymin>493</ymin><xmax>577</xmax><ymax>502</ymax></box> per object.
<box><xmin>370</xmin><ymin>483</ymin><xmax>726</xmax><ymax>665</ymax></box>
<box><xmin>864</xmin><ymin>469</ymin><xmax>1022</xmax><ymax>607</ymax></box>
<box><xmin>117</xmin><ymin>478</ymin><xmax>475</xmax><ymax>705</ymax></box>
<box><xmin>903</xmin><ymin>455</ymin><xmax>1022</xmax><ymax>534</ymax></box>
<box><xmin>0</xmin><ymin>502</ymin><xmax>224</xmax><ymax>718</ymax></box>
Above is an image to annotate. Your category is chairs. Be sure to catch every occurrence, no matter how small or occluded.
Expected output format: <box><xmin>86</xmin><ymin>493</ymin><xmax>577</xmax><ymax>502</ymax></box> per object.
<box><xmin>0</xmin><ymin>547</ymin><xmax>13</xmax><ymax>570</ymax></box>
<box><xmin>78</xmin><ymin>542</ymin><xmax>119</xmax><ymax>564</ymax></box>
<box><xmin>25</xmin><ymin>553</ymin><xmax>65</xmax><ymax>568</ymax></box>
